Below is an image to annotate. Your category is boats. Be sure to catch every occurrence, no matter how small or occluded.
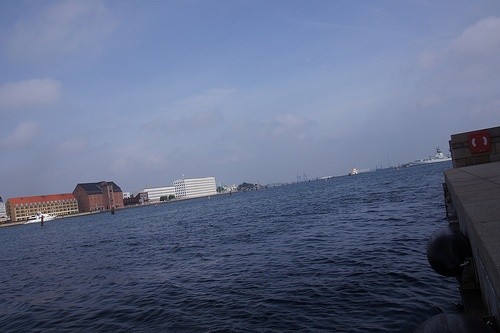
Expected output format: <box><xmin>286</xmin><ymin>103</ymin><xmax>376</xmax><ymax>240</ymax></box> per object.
<box><xmin>25</xmin><ymin>213</ymin><xmax>57</xmax><ymax>225</ymax></box>
